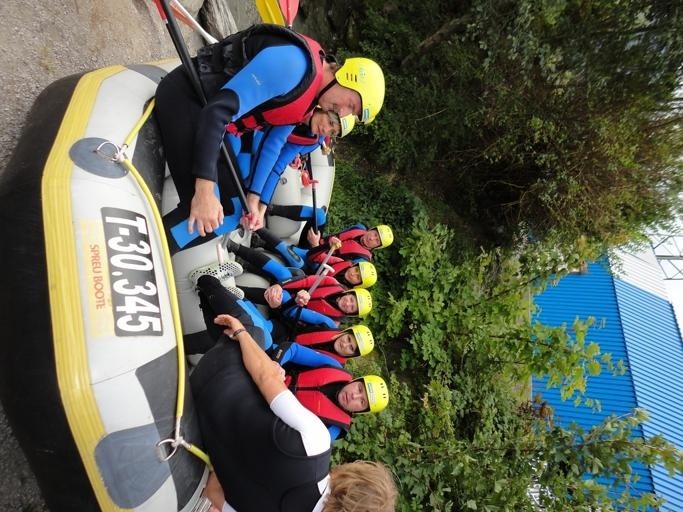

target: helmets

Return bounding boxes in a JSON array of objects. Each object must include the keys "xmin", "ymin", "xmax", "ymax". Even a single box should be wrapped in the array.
[
  {"xmin": 339, "ymin": 325, "xmax": 375, "ymax": 358},
  {"xmin": 352, "ymin": 261, "xmax": 378, "ymax": 288},
  {"xmin": 316, "ymin": 102, "xmax": 355, "ymax": 138},
  {"xmin": 348, "ymin": 375, "xmax": 389, "ymax": 414},
  {"xmin": 334, "ymin": 56, "xmax": 384, "ymax": 125},
  {"xmin": 368, "ymin": 224, "xmax": 394, "ymax": 249},
  {"xmin": 343, "ymin": 288, "xmax": 372, "ymax": 318}
]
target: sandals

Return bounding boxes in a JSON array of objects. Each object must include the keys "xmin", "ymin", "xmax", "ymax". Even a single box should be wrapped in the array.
[
  {"xmin": 188, "ymin": 261, "xmax": 243, "ymax": 287},
  {"xmin": 227, "ymin": 286, "xmax": 245, "ymax": 302}
]
